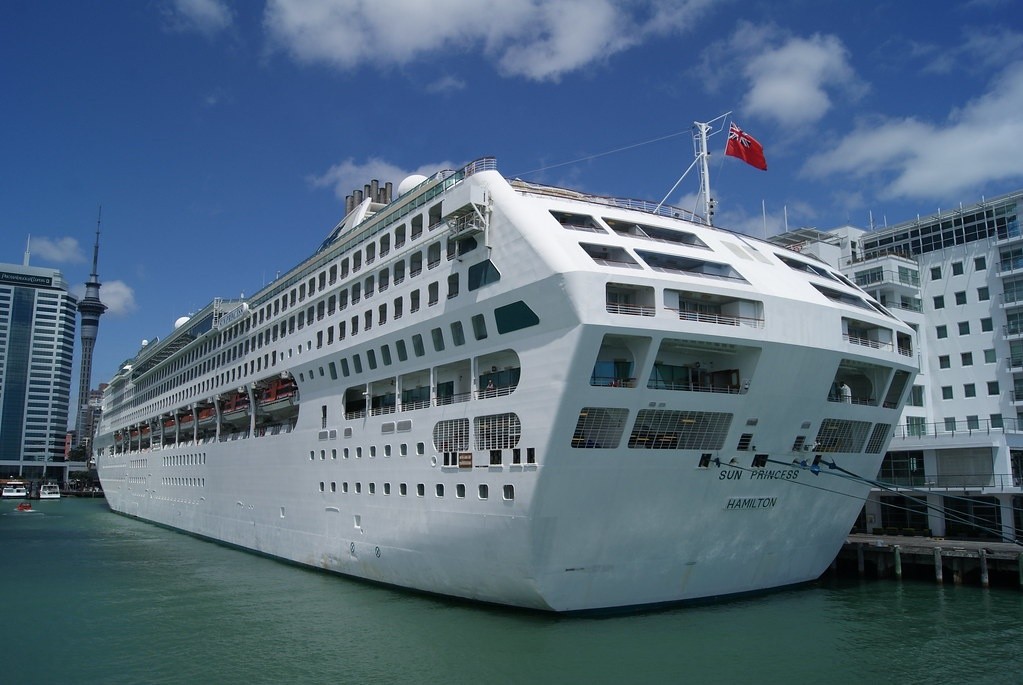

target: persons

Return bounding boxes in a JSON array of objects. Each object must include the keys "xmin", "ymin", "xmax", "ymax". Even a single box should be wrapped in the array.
[{"xmin": 486, "ymin": 380, "xmax": 494, "ymax": 389}]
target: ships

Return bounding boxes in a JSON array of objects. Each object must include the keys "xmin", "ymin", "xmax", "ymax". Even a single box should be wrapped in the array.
[{"xmin": 93, "ymin": 109, "xmax": 921, "ymax": 621}]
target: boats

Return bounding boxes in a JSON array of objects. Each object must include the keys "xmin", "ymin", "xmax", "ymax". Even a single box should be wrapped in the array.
[
  {"xmin": 197, "ymin": 408, "xmax": 218, "ymax": 430},
  {"xmin": 14, "ymin": 501, "xmax": 38, "ymax": 513},
  {"xmin": 165, "ymin": 420, "xmax": 177, "ymax": 435},
  {"xmin": 261, "ymin": 375, "xmax": 298, "ymax": 422},
  {"xmin": 114, "ymin": 433, "xmax": 122, "ymax": 447},
  {"xmin": 130, "ymin": 431, "xmax": 140, "ymax": 443},
  {"xmin": 1, "ymin": 481, "xmax": 29, "ymax": 498},
  {"xmin": 179, "ymin": 414, "xmax": 195, "ymax": 433},
  {"xmin": 142, "ymin": 427, "xmax": 150, "ymax": 442},
  {"xmin": 39, "ymin": 482, "xmax": 60, "ymax": 499},
  {"xmin": 219, "ymin": 392, "xmax": 252, "ymax": 429}
]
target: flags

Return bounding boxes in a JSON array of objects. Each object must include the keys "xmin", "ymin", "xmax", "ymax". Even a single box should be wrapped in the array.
[{"xmin": 724, "ymin": 120, "xmax": 768, "ymax": 171}]
